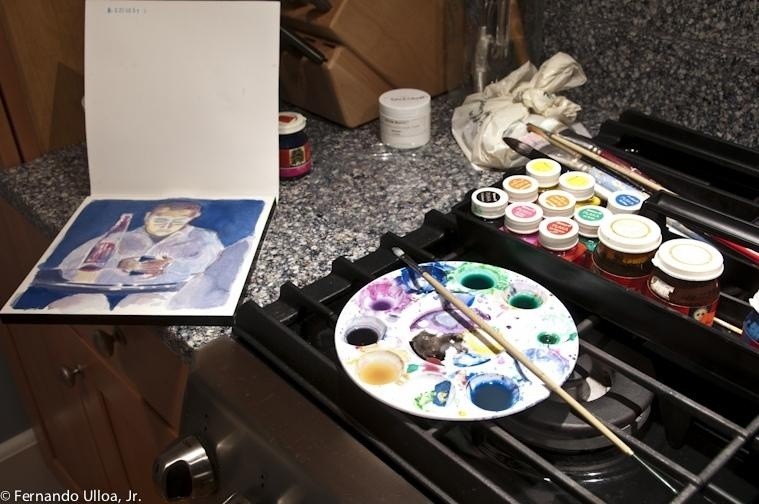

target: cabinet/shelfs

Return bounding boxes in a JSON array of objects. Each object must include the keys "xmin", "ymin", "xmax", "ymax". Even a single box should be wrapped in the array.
[
  {"xmin": 1, "ymin": 196, "xmax": 188, "ymax": 504},
  {"xmin": 0, "ymin": 0, "xmax": 85, "ymax": 172}
]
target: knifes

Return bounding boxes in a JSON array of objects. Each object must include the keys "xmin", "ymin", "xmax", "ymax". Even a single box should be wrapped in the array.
[{"xmin": 279, "ymin": 0, "xmax": 333, "ymax": 65}]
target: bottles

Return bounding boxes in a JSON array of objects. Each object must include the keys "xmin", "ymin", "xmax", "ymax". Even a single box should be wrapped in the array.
[
  {"xmin": 74, "ymin": 212, "xmax": 132, "ymax": 273},
  {"xmin": 375, "ymin": 84, "xmax": 434, "ymax": 152},
  {"xmin": 466, "ymin": 156, "xmax": 725, "ymax": 331},
  {"xmin": 276, "ymin": 108, "xmax": 316, "ymax": 181}
]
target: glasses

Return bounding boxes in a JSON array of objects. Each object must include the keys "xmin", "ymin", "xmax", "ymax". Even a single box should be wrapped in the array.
[{"xmin": 146, "ymin": 218, "xmax": 189, "ymax": 225}]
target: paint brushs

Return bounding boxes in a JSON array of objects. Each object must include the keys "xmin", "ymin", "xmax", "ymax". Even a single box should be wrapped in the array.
[
  {"xmin": 391, "ymin": 246, "xmax": 679, "ymax": 498},
  {"xmin": 501, "ymin": 123, "xmax": 759, "ymax": 267}
]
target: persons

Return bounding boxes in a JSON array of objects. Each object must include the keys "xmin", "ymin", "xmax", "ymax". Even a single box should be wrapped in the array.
[{"xmin": 43, "ymin": 200, "xmax": 252, "ymax": 313}]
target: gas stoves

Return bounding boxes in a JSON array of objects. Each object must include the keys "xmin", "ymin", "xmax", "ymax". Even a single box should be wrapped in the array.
[{"xmin": 150, "ymin": 105, "xmax": 758, "ymax": 502}]
